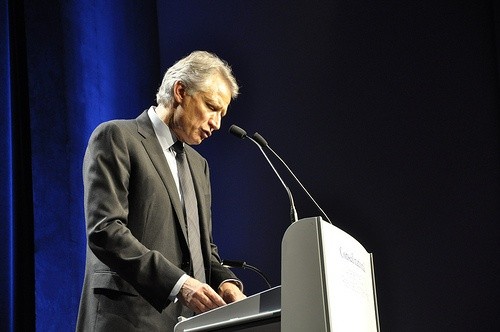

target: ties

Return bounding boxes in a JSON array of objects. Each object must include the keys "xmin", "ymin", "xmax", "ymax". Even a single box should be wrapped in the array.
[{"xmin": 170, "ymin": 140, "xmax": 206, "ymax": 284}]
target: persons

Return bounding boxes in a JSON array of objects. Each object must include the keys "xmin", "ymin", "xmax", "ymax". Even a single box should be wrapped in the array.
[{"xmin": 76, "ymin": 51, "xmax": 248, "ymax": 332}]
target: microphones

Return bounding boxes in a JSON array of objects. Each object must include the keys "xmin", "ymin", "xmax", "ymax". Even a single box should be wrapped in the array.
[
  {"xmin": 221, "ymin": 260, "xmax": 272, "ymax": 288},
  {"xmin": 229, "ymin": 125, "xmax": 332, "ymax": 223}
]
